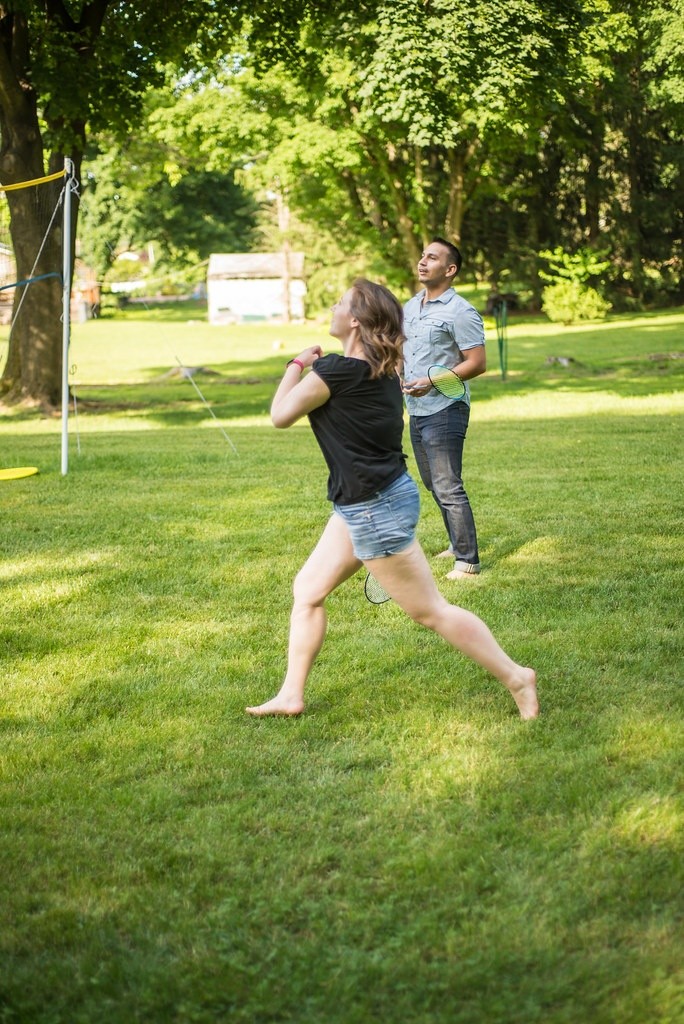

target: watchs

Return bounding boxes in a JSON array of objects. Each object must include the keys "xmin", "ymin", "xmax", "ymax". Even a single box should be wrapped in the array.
[{"xmin": 286, "ymin": 359, "xmax": 305, "ymax": 375}]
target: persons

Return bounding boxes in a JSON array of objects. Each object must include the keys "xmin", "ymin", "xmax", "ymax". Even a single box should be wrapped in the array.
[
  {"xmin": 396, "ymin": 237, "xmax": 488, "ymax": 581},
  {"xmin": 246, "ymin": 277, "xmax": 539, "ymax": 724}
]
